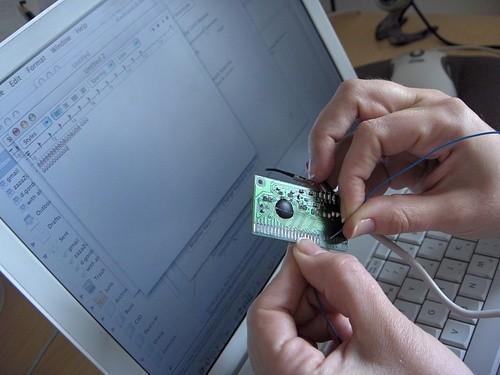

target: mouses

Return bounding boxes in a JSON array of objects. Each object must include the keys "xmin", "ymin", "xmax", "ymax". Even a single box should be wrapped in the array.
[{"xmin": 391, "ymin": 50, "xmax": 458, "ymax": 97}]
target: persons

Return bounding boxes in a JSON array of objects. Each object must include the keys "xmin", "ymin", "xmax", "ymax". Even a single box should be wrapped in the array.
[{"xmin": 245, "ymin": 79, "xmax": 500, "ymax": 375}]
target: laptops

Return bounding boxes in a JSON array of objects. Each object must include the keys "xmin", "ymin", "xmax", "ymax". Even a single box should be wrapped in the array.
[{"xmin": 0, "ymin": 0, "xmax": 500, "ymax": 375}]
